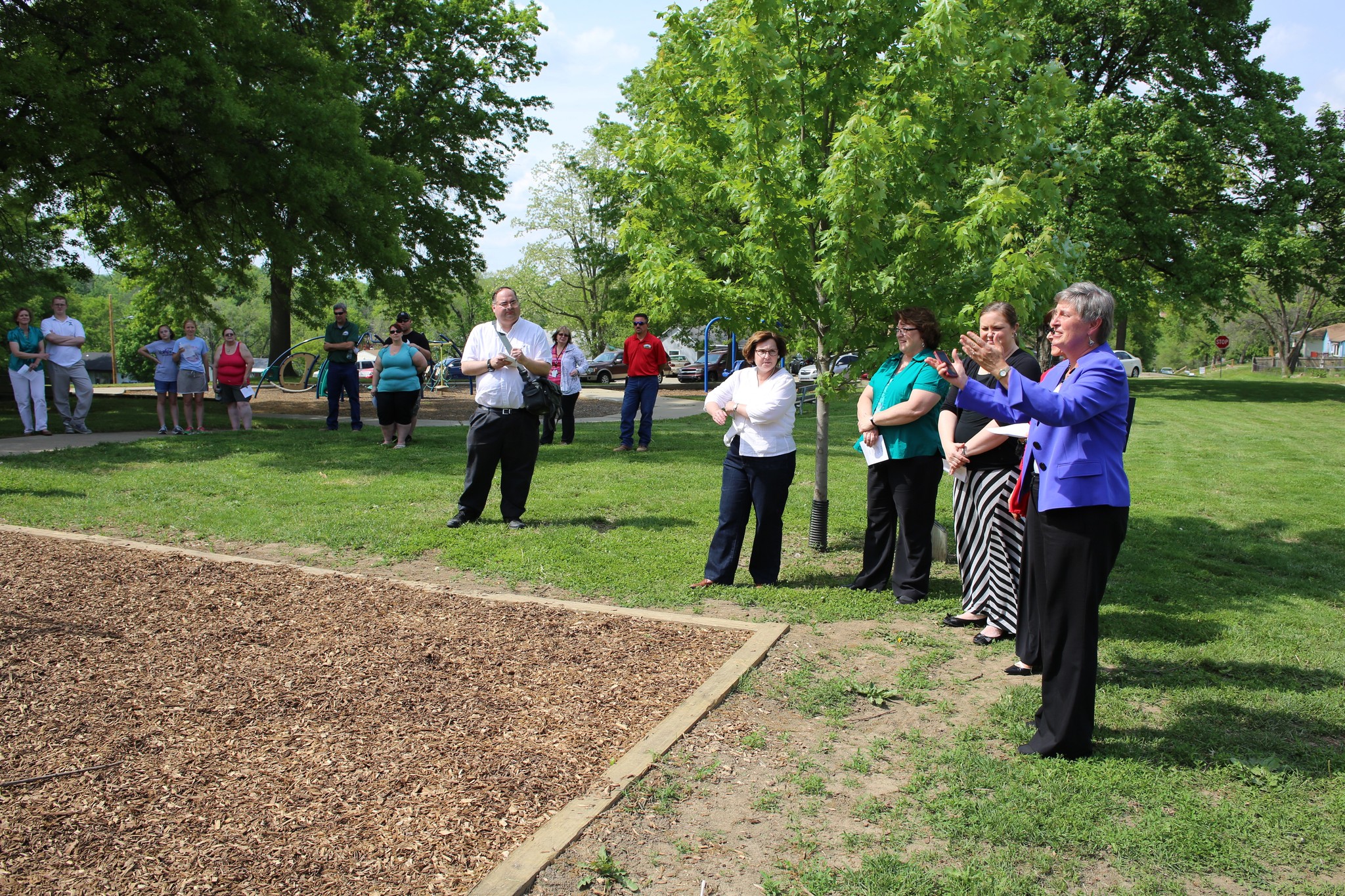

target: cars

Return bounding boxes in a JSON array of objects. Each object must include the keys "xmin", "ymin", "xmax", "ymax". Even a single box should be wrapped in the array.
[
  {"xmin": 1159, "ymin": 368, "xmax": 1174, "ymax": 375},
  {"xmin": 1109, "ymin": 350, "xmax": 1142, "ymax": 378},
  {"xmin": 356, "ymin": 360, "xmax": 377, "ymax": 380},
  {"xmin": 797, "ymin": 353, "xmax": 859, "ymax": 383},
  {"xmin": 661, "ymin": 355, "xmax": 691, "ymax": 377},
  {"xmin": 789, "ymin": 351, "xmax": 817, "ymax": 374},
  {"xmin": 431, "ymin": 358, "xmax": 477, "ymax": 380},
  {"xmin": 578, "ymin": 350, "xmax": 663, "ymax": 385}
]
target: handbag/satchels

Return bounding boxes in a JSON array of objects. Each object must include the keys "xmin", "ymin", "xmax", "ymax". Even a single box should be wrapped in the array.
[{"xmin": 522, "ymin": 377, "xmax": 563, "ymax": 432}]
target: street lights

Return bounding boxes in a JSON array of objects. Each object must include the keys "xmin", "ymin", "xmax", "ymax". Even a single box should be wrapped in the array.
[{"xmin": 107, "ymin": 294, "xmax": 134, "ymax": 384}]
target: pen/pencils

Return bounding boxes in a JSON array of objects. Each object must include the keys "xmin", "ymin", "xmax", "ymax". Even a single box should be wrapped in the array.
[{"xmin": 521, "ymin": 345, "xmax": 526, "ymax": 349}]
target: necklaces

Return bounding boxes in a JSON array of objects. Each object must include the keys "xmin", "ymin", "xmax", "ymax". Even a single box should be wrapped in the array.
[
  {"xmin": 758, "ymin": 366, "xmax": 776, "ymax": 388},
  {"xmin": 1061, "ymin": 363, "xmax": 1077, "ymax": 384},
  {"xmin": 227, "ymin": 349, "xmax": 230, "ymax": 352}
]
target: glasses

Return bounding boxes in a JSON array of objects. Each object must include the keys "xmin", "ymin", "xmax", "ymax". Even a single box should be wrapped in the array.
[
  {"xmin": 334, "ymin": 311, "xmax": 345, "ymax": 315},
  {"xmin": 894, "ymin": 328, "xmax": 917, "ymax": 334},
  {"xmin": 497, "ymin": 301, "xmax": 520, "ymax": 309},
  {"xmin": 53, "ymin": 303, "xmax": 66, "ymax": 306},
  {"xmin": 558, "ymin": 333, "xmax": 569, "ymax": 337},
  {"xmin": 1047, "ymin": 328, "xmax": 1055, "ymax": 335},
  {"xmin": 397, "ymin": 317, "xmax": 409, "ymax": 323},
  {"xmin": 633, "ymin": 321, "xmax": 647, "ymax": 326},
  {"xmin": 755, "ymin": 349, "xmax": 780, "ymax": 356},
  {"xmin": 225, "ymin": 334, "xmax": 235, "ymax": 337},
  {"xmin": 390, "ymin": 329, "xmax": 401, "ymax": 334}
]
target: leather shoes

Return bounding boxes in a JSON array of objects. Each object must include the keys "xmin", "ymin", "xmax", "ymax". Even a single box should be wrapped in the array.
[
  {"xmin": 1004, "ymin": 661, "xmax": 1032, "ymax": 675},
  {"xmin": 841, "ymin": 584, "xmax": 868, "ymax": 591},
  {"xmin": 894, "ymin": 596, "xmax": 918, "ymax": 605},
  {"xmin": 755, "ymin": 582, "xmax": 764, "ymax": 589},
  {"xmin": 690, "ymin": 578, "xmax": 717, "ymax": 588},
  {"xmin": 972, "ymin": 626, "xmax": 1016, "ymax": 646},
  {"xmin": 942, "ymin": 612, "xmax": 988, "ymax": 627}
]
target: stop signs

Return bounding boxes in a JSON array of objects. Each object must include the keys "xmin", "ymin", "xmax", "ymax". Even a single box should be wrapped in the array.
[{"xmin": 1215, "ymin": 335, "xmax": 1229, "ymax": 349}]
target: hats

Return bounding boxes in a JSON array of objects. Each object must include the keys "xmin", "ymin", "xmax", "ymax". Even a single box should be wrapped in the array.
[{"xmin": 396, "ymin": 311, "xmax": 410, "ymax": 321}]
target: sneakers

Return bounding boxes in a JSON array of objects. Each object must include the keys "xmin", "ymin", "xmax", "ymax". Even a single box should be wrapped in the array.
[
  {"xmin": 68, "ymin": 423, "xmax": 92, "ymax": 434},
  {"xmin": 196, "ymin": 426, "xmax": 208, "ymax": 433},
  {"xmin": 613, "ymin": 444, "xmax": 633, "ymax": 451},
  {"xmin": 636, "ymin": 445, "xmax": 648, "ymax": 452},
  {"xmin": 503, "ymin": 517, "xmax": 527, "ymax": 529},
  {"xmin": 64, "ymin": 425, "xmax": 75, "ymax": 434},
  {"xmin": 172, "ymin": 425, "xmax": 184, "ymax": 435},
  {"xmin": 446, "ymin": 511, "xmax": 481, "ymax": 527},
  {"xmin": 158, "ymin": 425, "xmax": 168, "ymax": 434},
  {"xmin": 183, "ymin": 426, "xmax": 195, "ymax": 435}
]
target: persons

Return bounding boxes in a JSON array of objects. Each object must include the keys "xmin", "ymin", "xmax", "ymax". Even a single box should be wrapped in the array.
[
  {"xmin": 7, "ymin": 307, "xmax": 52, "ymax": 436},
  {"xmin": 690, "ymin": 331, "xmax": 796, "ymax": 589},
  {"xmin": 212, "ymin": 327, "xmax": 255, "ymax": 431},
  {"xmin": 540, "ymin": 326, "xmax": 589, "ymax": 445},
  {"xmin": 924, "ymin": 282, "xmax": 1131, "ymax": 760},
  {"xmin": 40, "ymin": 296, "xmax": 94, "ymax": 434},
  {"xmin": 381, "ymin": 311, "xmax": 432, "ymax": 442},
  {"xmin": 839, "ymin": 306, "xmax": 951, "ymax": 605},
  {"xmin": 370, "ymin": 323, "xmax": 428, "ymax": 449},
  {"xmin": 319, "ymin": 303, "xmax": 364, "ymax": 432},
  {"xmin": 614, "ymin": 313, "xmax": 667, "ymax": 452},
  {"xmin": 172, "ymin": 320, "xmax": 210, "ymax": 435},
  {"xmin": 447, "ymin": 286, "xmax": 552, "ymax": 528},
  {"xmin": 138, "ymin": 324, "xmax": 185, "ymax": 434}
]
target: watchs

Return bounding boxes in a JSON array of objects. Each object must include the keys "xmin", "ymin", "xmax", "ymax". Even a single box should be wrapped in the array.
[
  {"xmin": 869, "ymin": 415, "xmax": 877, "ymax": 427},
  {"xmin": 487, "ymin": 359, "xmax": 495, "ymax": 372},
  {"xmin": 957, "ymin": 447, "xmax": 968, "ymax": 458},
  {"xmin": 995, "ymin": 366, "xmax": 1012, "ymax": 380},
  {"xmin": 732, "ymin": 403, "xmax": 739, "ymax": 413}
]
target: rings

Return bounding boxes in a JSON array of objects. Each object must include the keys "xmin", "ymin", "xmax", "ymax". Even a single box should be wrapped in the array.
[{"xmin": 972, "ymin": 351, "xmax": 976, "ymax": 356}]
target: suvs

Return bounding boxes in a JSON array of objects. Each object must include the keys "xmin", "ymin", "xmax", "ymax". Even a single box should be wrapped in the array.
[{"xmin": 677, "ymin": 348, "xmax": 746, "ymax": 383}]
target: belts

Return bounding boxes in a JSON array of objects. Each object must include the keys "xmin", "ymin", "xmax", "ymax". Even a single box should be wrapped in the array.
[{"xmin": 479, "ymin": 405, "xmax": 527, "ymax": 415}]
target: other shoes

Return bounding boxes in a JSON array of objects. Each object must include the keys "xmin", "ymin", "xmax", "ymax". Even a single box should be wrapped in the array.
[
  {"xmin": 318, "ymin": 427, "xmax": 335, "ymax": 431},
  {"xmin": 40, "ymin": 429, "xmax": 52, "ymax": 436},
  {"xmin": 351, "ymin": 429, "xmax": 359, "ymax": 432},
  {"xmin": 375, "ymin": 435, "xmax": 413, "ymax": 450},
  {"xmin": 1026, "ymin": 721, "xmax": 1037, "ymax": 731},
  {"xmin": 24, "ymin": 430, "xmax": 37, "ymax": 436},
  {"xmin": 1018, "ymin": 743, "xmax": 1035, "ymax": 755}
]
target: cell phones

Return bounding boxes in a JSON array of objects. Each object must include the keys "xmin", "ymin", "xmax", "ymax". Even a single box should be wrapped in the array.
[{"xmin": 934, "ymin": 351, "xmax": 958, "ymax": 377}]
